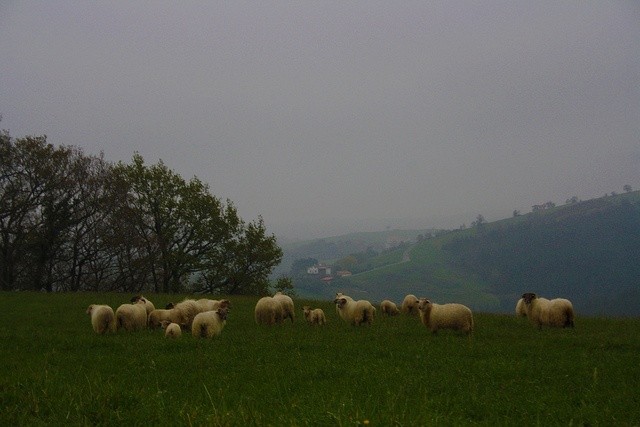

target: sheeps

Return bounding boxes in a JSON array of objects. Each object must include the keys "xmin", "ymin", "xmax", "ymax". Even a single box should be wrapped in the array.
[
  {"xmin": 334, "ymin": 292, "xmax": 377, "ymax": 328},
  {"xmin": 414, "ymin": 297, "xmax": 474, "ymax": 338},
  {"xmin": 514, "ymin": 292, "xmax": 546, "ymax": 326},
  {"xmin": 254, "ymin": 291, "xmax": 296, "ymax": 328},
  {"xmin": 84, "ymin": 302, "xmax": 115, "ymax": 337},
  {"xmin": 128, "ymin": 294, "xmax": 233, "ymax": 342},
  {"xmin": 300, "ymin": 305, "xmax": 330, "ymax": 328},
  {"xmin": 380, "ymin": 299, "xmax": 400, "ymax": 320},
  {"xmin": 401, "ymin": 294, "xmax": 419, "ymax": 317},
  {"xmin": 115, "ymin": 298, "xmax": 148, "ymax": 335},
  {"xmin": 521, "ymin": 292, "xmax": 574, "ymax": 332}
]
